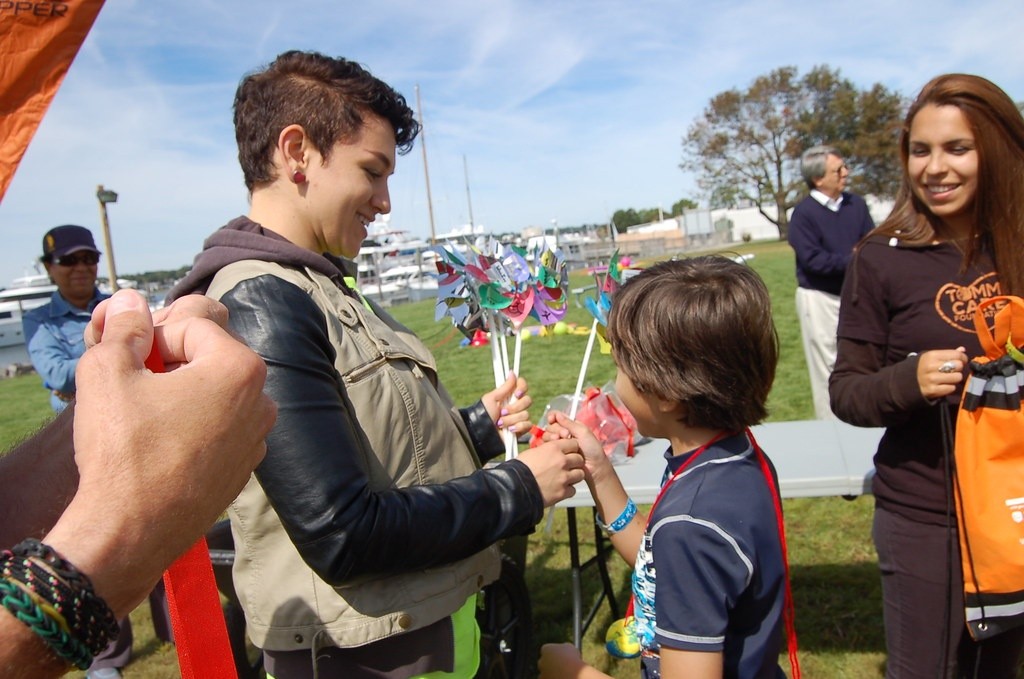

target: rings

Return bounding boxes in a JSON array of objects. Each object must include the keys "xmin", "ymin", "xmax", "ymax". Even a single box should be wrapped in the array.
[{"xmin": 938, "ymin": 360, "xmax": 957, "ymax": 374}]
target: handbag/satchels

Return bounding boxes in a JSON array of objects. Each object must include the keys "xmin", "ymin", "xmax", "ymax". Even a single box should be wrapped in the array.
[{"xmin": 948, "ymin": 283, "xmax": 1024, "ymax": 641}]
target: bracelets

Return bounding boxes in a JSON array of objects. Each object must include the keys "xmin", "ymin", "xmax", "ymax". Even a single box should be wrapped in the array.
[
  {"xmin": 0, "ymin": 536, "xmax": 124, "ymax": 670},
  {"xmin": 594, "ymin": 497, "xmax": 637, "ymax": 540}
]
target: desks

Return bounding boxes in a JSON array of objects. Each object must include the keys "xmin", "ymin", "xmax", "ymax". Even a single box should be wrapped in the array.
[{"xmin": 546, "ymin": 418, "xmax": 887, "ymax": 654}]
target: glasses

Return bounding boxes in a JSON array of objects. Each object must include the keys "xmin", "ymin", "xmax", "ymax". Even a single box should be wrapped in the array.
[
  {"xmin": 827, "ymin": 160, "xmax": 849, "ymax": 175},
  {"xmin": 40, "ymin": 256, "xmax": 100, "ymax": 266}
]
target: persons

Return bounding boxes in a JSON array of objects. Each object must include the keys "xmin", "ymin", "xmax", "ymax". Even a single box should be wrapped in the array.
[
  {"xmin": 786, "ymin": 143, "xmax": 878, "ymax": 502},
  {"xmin": 535, "ymin": 252, "xmax": 789, "ymax": 679},
  {"xmin": 827, "ymin": 71, "xmax": 1024, "ymax": 679},
  {"xmin": 161, "ymin": 48, "xmax": 588, "ymax": 678},
  {"xmin": 21, "ymin": 225, "xmax": 117, "ymax": 422},
  {"xmin": 0, "ymin": 287, "xmax": 279, "ymax": 679}
]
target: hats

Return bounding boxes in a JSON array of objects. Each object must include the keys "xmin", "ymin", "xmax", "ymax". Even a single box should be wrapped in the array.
[{"xmin": 43, "ymin": 225, "xmax": 102, "ymax": 257}]
